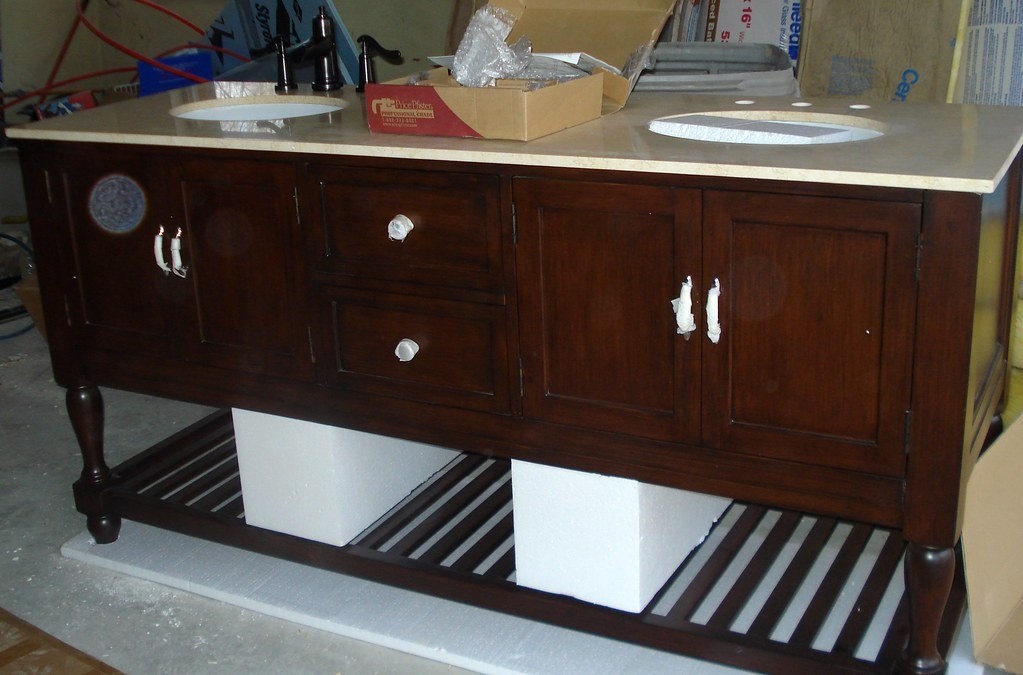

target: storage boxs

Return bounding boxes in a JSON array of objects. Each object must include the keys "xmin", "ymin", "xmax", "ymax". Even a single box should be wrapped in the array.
[
  {"xmin": 365, "ymin": 0, "xmax": 676, "ymax": 142},
  {"xmin": 231, "ymin": 407, "xmax": 463, "ymax": 546},
  {"xmin": 511, "ymin": 459, "xmax": 734, "ymax": 615}
]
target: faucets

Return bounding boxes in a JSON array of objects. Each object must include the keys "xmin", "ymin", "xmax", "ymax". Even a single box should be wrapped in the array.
[{"xmin": 277, "ymin": 6, "xmax": 344, "ymax": 92}]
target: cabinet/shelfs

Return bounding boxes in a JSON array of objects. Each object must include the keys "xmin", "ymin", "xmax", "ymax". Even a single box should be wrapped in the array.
[{"xmin": 3, "ymin": 79, "xmax": 1023, "ymax": 675}]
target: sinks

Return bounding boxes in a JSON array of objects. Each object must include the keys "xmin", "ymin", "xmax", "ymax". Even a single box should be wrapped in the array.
[
  {"xmin": 646, "ymin": 110, "xmax": 889, "ymax": 145},
  {"xmin": 168, "ymin": 95, "xmax": 350, "ymax": 122}
]
